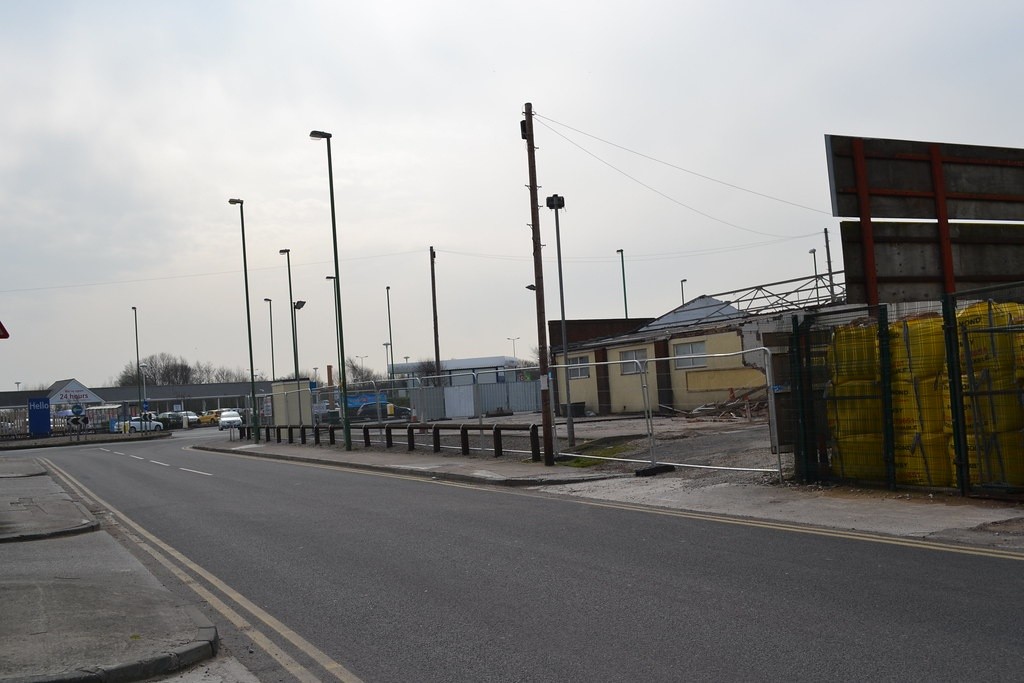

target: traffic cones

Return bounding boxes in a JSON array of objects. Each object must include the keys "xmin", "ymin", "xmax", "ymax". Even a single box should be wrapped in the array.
[{"xmin": 410, "ymin": 403, "xmax": 420, "ymax": 423}]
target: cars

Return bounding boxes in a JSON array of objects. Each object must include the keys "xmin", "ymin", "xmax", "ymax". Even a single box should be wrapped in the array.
[
  {"xmin": 217, "ymin": 411, "xmax": 243, "ymax": 431},
  {"xmin": 136, "ymin": 406, "xmax": 259, "ymax": 430},
  {"xmin": 313, "ymin": 404, "xmax": 340, "ymax": 424},
  {"xmin": 113, "ymin": 416, "xmax": 164, "ymax": 434},
  {"xmin": 357, "ymin": 401, "xmax": 411, "ymax": 422}
]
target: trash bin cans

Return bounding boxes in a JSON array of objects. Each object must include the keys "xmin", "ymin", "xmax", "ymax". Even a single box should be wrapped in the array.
[{"xmin": 561, "ymin": 401, "xmax": 585, "ymax": 417}]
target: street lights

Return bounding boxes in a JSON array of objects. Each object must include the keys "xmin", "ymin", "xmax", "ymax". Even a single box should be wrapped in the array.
[
  {"xmin": 278, "ymin": 247, "xmax": 297, "ymax": 380},
  {"xmin": 403, "ymin": 356, "xmax": 410, "ymax": 363},
  {"xmin": 130, "ymin": 305, "xmax": 142, "ymax": 413},
  {"xmin": 264, "ymin": 297, "xmax": 275, "ymax": 383},
  {"xmin": 14, "ymin": 381, "xmax": 22, "ymax": 391},
  {"xmin": 140, "ymin": 362, "xmax": 148, "ymax": 412},
  {"xmin": 226, "ymin": 196, "xmax": 260, "ymax": 426},
  {"xmin": 615, "ymin": 248, "xmax": 628, "ymax": 319},
  {"xmin": 323, "ymin": 274, "xmax": 344, "ymax": 424},
  {"xmin": 292, "ymin": 301, "xmax": 308, "ymax": 424},
  {"xmin": 308, "ymin": 128, "xmax": 354, "ymax": 450},
  {"xmin": 506, "ymin": 337, "xmax": 521, "ymax": 381},
  {"xmin": 680, "ymin": 279, "xmax": 687, "ymax": 306},
  {"xmin": 545, "ymin": 193, "xmax": 577, "ymax": 447},
  {"xmin": 525, "ymin": 284, "xmax": 556, "ymax": 466},
  {"xmin": 312, "ymin": 367, "xmax": 319, "ymax": 388},
  {"xmin": 355, "ymin": 355, "xmax": 369, "ymax": 381},
  {"xmin": 383, "ymin": 341, "xmax": 391, "ymax": 379},
  {"xmin": 809, "ymin": 248, "xmax": 820, "ymax": 305},
  {"xmin": 385, "ymin": 286, "xmax": 396, "ymax": 388}
]
